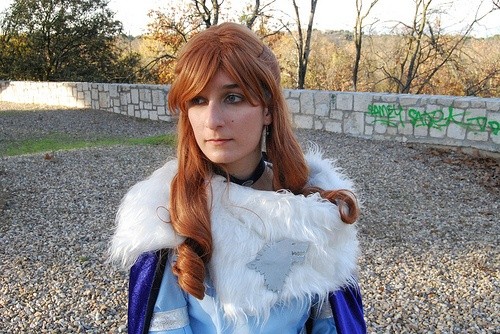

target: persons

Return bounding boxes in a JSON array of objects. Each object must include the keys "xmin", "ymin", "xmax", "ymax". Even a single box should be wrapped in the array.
[{"xmin": 120, "ymin": 22, "xmax": 371, "ymax": 334}]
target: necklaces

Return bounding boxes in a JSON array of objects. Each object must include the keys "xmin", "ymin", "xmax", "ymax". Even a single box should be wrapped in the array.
[{"xmin": 210, "ymin": 155, "xmax": 268, "ymax": 189}]
